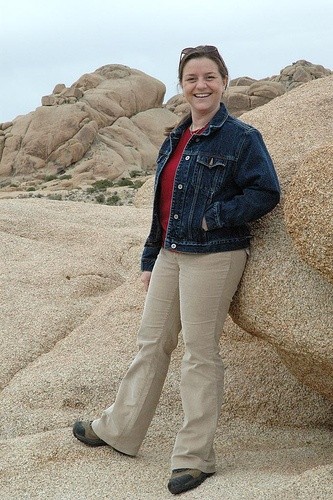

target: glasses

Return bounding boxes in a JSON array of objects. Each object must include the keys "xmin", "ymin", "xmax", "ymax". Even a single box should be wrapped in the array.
[{"xmin": 178, "ymin": 45, "xmax": 225, "ymax": 66}]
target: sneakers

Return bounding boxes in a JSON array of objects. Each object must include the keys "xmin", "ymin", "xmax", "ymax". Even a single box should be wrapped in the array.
[
  {"xmin": 168, "ymin": 469, "xmax": 215, "ymax": 495},
  {"xmin": 72, "ymin": 420, "xmax": 134, "ymax": 456}
]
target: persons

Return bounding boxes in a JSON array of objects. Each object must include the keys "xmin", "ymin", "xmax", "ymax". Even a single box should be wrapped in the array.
[{"xmin": 72, "ymin": 45, "xmax": 282, "ymax": 494}]
